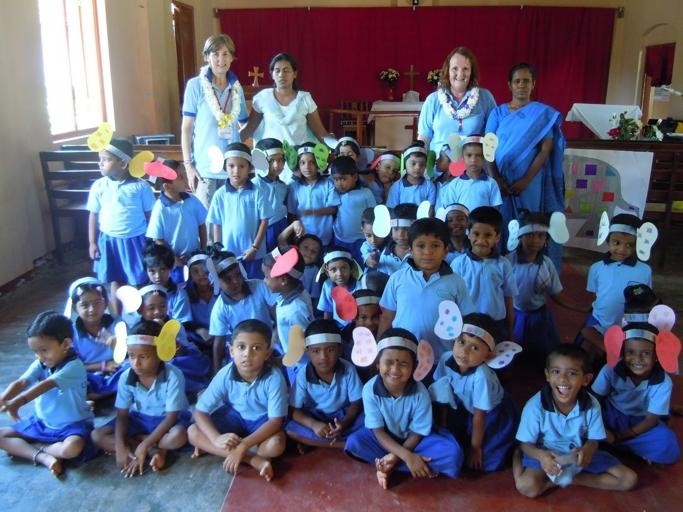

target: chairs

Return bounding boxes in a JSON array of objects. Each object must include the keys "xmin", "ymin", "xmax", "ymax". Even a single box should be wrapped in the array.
[{"xmin": 39, "ymin": 150, "xmax": 103, "ymax": 261}]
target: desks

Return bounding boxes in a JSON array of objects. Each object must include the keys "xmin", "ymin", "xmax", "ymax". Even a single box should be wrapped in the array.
[{"xmin": 367, "ymin": 99, "xmax": 427, "ymax": 149}]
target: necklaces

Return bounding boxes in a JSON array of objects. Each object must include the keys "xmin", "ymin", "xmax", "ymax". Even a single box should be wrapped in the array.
[
  {"xmin": 200, "ymin": 65, "xmax": 241, "ymax": 128},
  {"xmin": 438, "ymin": 85, "xmax": 480, "ymax": 119}
]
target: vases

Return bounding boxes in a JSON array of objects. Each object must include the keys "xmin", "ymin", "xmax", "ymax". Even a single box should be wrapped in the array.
[{"xmin": 382, "ymin": 86, "xmax": 398, "ymax": 100}]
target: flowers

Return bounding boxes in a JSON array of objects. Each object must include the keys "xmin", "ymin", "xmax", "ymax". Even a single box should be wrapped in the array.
[
  {"xmin": 428, "ymin": 69, "xmax": 443, "ymax": 83},
  {"xmin": 377, "ymin": 67, "xmax": 403, "ymax": 87}
]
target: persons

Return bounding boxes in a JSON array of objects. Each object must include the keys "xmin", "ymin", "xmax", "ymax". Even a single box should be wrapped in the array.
[
  {"xmin": 418, "ymin": 45, "xmax": 498, "ymax": 177},
  {"xmin": 240, "ymin": 52, "xmax": 331, "ymax": 186},
  {"xmin": 0, "ymin": 133, "xmax": 681, "ymax": 499},
  {"xmin": 179, "ymin": 33, "xmax": 250, "ymax": 209},
  {"xmin": 486, "ymin": 62, "xmax": 566, "ymax": 275}
]
